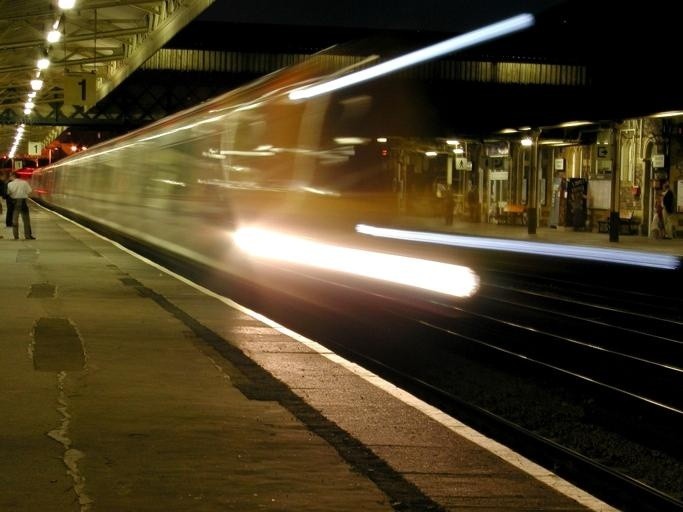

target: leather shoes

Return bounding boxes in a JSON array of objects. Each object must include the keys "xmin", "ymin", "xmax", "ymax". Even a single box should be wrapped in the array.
[{"xmin": 26, "ymin": 235, "xmax": 36, "ymax": 239}]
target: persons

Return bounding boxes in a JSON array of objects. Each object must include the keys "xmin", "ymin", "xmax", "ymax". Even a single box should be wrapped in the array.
[
  {"xmin": 0, "ymin": 171, "xmax": 39, "ymax": 240},
  {"xmin": 660, "ymin": 183, "xmax": 675, "ymax": 242},
  {"xmin": 468, "ymin": 184, "xmax": 479, "ymax": 219},
  {"xmin": 443, "ymin": 183, "xmax": 456, "ymax": 224}
]
